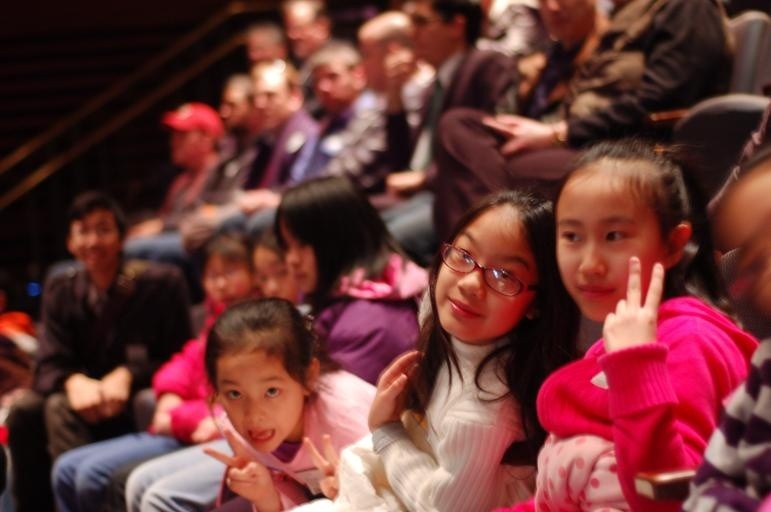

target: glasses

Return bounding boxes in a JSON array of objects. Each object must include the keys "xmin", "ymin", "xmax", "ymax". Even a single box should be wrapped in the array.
[{"xmin": 439, "ymin": 240, "xmax": 537, "ymax": 298}]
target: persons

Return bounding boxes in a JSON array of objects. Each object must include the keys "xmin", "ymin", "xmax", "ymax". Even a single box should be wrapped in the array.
[{"xmin": 0, "ymin": 1, "xmax": 771, "ymax": 512}]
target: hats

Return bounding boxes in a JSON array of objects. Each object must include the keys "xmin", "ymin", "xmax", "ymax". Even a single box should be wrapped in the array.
[{"xmin": 162, "ymin": 101, "xmax": 224, "ymax": 136}]
